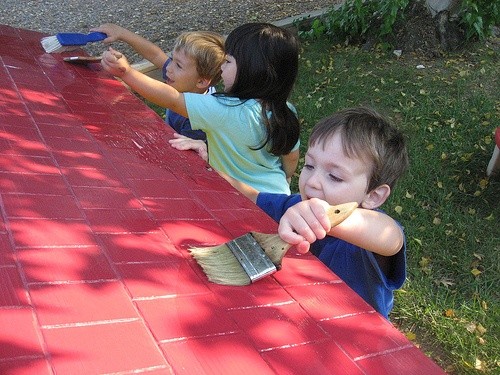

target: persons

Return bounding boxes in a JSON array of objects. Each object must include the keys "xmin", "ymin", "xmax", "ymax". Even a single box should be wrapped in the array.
[
  {"xmin": 168, "ymin": 106, "xmax": 409, "ymax": 320},
  {"xmin": 88, "ymin": 21, "xmax": 228, "ymax": 164},
  {"xmin": 99, "ymin": 22, "xmax": 300, "ymax": 197}
]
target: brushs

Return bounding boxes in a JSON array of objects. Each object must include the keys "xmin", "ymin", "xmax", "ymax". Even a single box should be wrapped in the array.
[
  {"xmin": 40, "ymin": 31, "xmax": 109, "ymax": 54},
  {"xmin": 186, "ymin": 201, "xmax": 360, "ymax": 288},
  {"xmin": 62, "ymin": 53, "xmax": 122, "ymax": 63}
]
